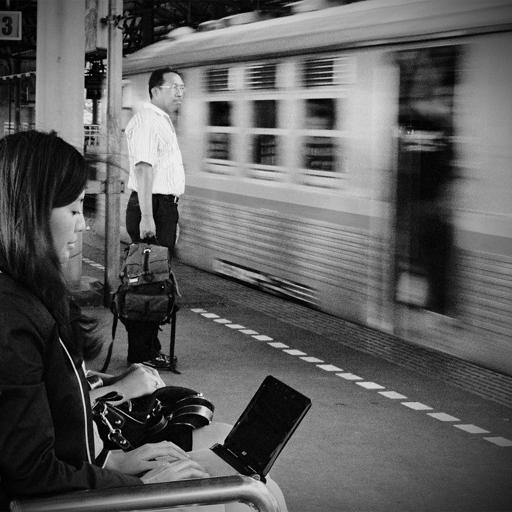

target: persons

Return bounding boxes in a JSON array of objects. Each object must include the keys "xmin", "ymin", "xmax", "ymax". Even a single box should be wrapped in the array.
[
  {"xmin": 0, "ymin": 128, "xmax": 289, "ymax": 512},
  {"xmin": 79, "ymin": 360, "xmax": 235, "ymax": 450},
  {"xmin": 123, "ymin": 68, "xmax": 188, "ymax": 372}
]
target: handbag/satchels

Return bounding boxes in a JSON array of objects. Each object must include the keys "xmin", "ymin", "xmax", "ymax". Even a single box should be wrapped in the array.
[{"xmin": 93, "ymin": 378, "xmax": 217, "ymax": 452}]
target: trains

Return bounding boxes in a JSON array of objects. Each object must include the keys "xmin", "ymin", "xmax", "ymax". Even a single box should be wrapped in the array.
[{"xmin": 0, "ymin": 0, "xmax": 512, "ymax": 374}]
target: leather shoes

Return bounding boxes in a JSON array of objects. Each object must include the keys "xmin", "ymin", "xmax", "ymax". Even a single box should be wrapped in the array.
[{"xmin": 127, "ymin": 352, "xmax": 178, "ymax": 371}]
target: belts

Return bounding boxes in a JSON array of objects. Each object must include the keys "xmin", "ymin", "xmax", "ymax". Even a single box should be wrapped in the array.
[{"xmin": 130, "ymin": 191, "xmax": 178, "ymax": 205}]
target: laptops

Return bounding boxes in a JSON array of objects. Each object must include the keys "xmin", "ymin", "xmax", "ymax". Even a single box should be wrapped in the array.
[{"xmin": 154, "ymin": 374, "xmax": 313, "ymax": 485}]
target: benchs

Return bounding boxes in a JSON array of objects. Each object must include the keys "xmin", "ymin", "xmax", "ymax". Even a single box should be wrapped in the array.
[{"xmin": 6, "ymin": 472, "xmax": 284, "ymax": 512}]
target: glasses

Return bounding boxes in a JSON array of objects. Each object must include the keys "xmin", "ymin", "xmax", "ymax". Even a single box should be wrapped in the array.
[{"xmin": 157, "ymin": 84, "xmax": 185, "ymax": 92}]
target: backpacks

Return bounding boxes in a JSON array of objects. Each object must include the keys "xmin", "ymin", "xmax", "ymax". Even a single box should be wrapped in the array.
[{"xmin": 109, "ymin": 232, "xmax": 186, "ymax": 329}]
